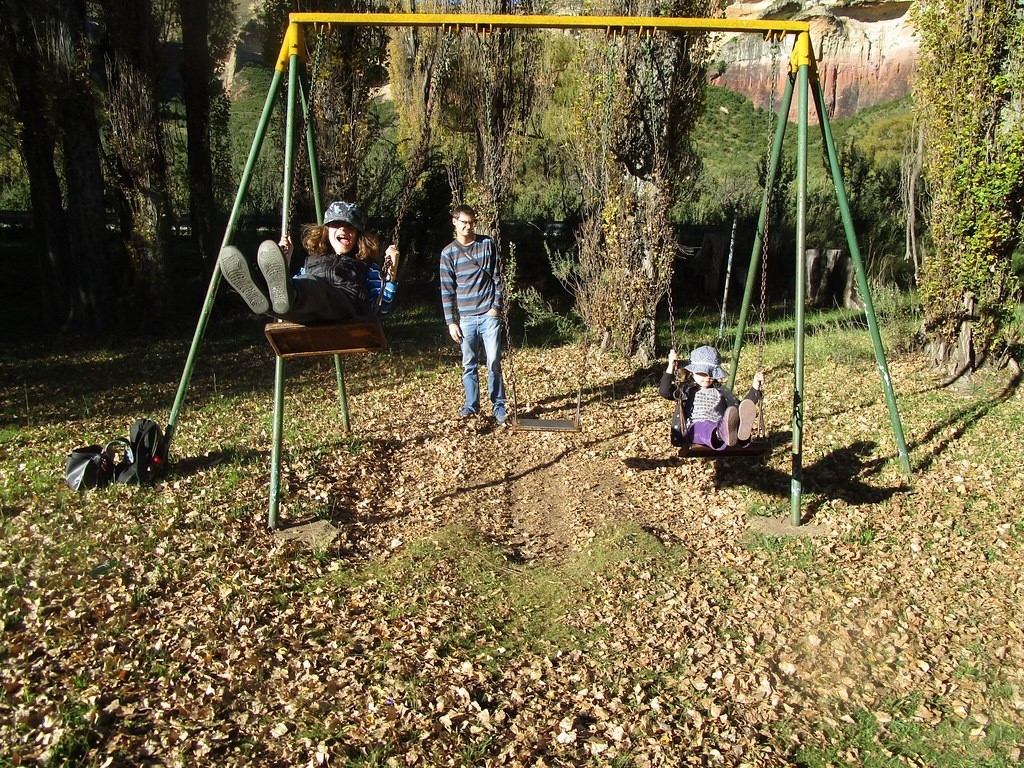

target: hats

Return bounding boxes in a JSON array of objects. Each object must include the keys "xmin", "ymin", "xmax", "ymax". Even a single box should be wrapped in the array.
[
  {"xmin": 323, "ymin": 199, "xmax": 367, "ymax": 233},
  {"xmin": 682, "ymin": 346, "xmax": 730, "ymax": 380}
]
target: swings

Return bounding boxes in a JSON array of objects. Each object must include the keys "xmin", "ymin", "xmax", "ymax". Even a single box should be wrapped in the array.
[
  {"xmin": 481, "ymin": 28, "xmax": 619, "ymax": 432},
  {"xmin": 648, "ymin": 29, "xmax": 773, "ymax": 459},
  {"xmin": 264, "ymin": 27, "xmax": 456, "ymax": 358}
]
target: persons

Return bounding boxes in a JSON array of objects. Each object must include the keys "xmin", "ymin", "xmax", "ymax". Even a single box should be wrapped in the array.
[
  {"xmin": 660, "ymin": 346, "xmax": 765, "ymax": 450},
  {"xmin": 440, "ymin": 205, "xmax": 508, "ymax": 423},
  {"xmin": 220, "ymin": 201, "xmax": 398, "ymax": 326}
]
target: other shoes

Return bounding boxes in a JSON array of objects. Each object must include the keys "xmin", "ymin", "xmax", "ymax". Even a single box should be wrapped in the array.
[
  {"xmin": 220, "ymin": 245, "xmax": 270, "ymax": 315},
  {"xmin": 257, "ymin": 239, "xmax": 290, "ymax": 315}
]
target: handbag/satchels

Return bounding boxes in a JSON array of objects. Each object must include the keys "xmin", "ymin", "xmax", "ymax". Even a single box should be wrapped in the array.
[{"xmin": 64, "ymin": 437, "xmax": 134, "ymax": 494}]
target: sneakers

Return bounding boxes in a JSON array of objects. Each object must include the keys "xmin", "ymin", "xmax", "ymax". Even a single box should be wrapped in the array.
[
  {"xmin": 716, "ymin": 406, "xmax": 739, "ymax": 447},
  {"xmin": 737, "ymin": 399, "xmax": 756, "ymax": 441},
  {"xmin": 456, "ymin": 408, "xmax": 480, "ymax": 418},
  {"xmin": 492, "ymin": 405, "xmax": 508, "ymax": 424}
]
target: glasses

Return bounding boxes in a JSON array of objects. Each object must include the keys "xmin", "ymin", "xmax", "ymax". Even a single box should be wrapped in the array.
[{"xmin": 457, "ymin": 219, "xmax": 474, "ymax": 225}]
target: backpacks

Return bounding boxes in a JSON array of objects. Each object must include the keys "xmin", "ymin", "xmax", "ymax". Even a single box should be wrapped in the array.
[{"xmin": 112, "ymin": 418, "xmax": 169, "ymax": 487}]
